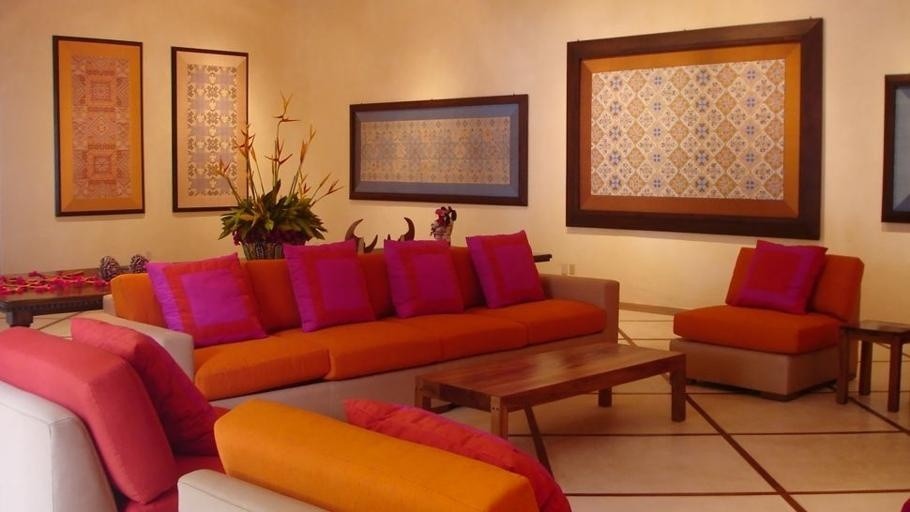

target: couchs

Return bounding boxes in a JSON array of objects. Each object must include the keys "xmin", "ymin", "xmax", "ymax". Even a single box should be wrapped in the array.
[{"xmin": 68, "ymin": 265, "xmax": 622, "ymax": 429}]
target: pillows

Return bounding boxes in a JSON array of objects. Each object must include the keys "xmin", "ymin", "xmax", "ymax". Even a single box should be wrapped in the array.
[
  {"xmin": 462, "ymin": 228, "xmax": 549, "ymax": 311},
  {"xmin": 727, "ymin": 238, "xmax": 831, "ymax": 317},
  {"xmin": 65, "ymin": 314, "xmax": 221, "ymax": 448},
  {"xmin": 281, "ymin": 236, "xmax": 379, "ymax": 334},
  {"xmin": 340, "ymin": 395, "xmax": 575, "ymax": 512},
  {"xmin": 379, "ymin": 235, "xmax": 468, "ymax": 319},
  {"xmin": 141, "ymin": 251, "xmax": 269, "ymax": 350}
]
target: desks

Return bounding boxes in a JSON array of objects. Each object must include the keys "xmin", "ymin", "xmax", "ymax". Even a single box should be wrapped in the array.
[
  {"xmin": 833, "ymin": 315, "xmax": 910, "ymax": 413},
  {"xmin": 0, "ymin": 265, "xmax": 136, "ymax": 332}
]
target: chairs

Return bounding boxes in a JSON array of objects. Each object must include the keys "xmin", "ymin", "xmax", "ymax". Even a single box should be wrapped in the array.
[
  {"xmin": 172, "ymin": 394, "xmax": 546, "ymax": 511},
  {"xmin": 666, "ymin": 244, "xmax": 867, "ymax": 403},
  {"xmin": 0, "ymin": 326, "xmax": 231, "ymax": 511}
]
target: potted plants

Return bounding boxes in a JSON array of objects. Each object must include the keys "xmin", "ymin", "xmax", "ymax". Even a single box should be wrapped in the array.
[{"xmin": 207, "ymin": 84, "xmax": 345, "ymax": 265}]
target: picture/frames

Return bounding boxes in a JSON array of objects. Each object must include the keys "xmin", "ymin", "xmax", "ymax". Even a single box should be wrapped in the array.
[
  {"xmin": 564, "ymin": 18, "xmax": 825, "ymax": 244},
  {"xmin": 343, "ymin": 93, "xmax": 529, "ymax": 208},
  {"xmin": 168, "ymin": 44, "xmax": 251, "ymax": 213},
  {"xmin": 50, "ymin": 33, "xmax": 146, "ymax": 218},
  {"xmin": 880, "ymin": 72, "xmax": 910, "ymax": 225}
]
